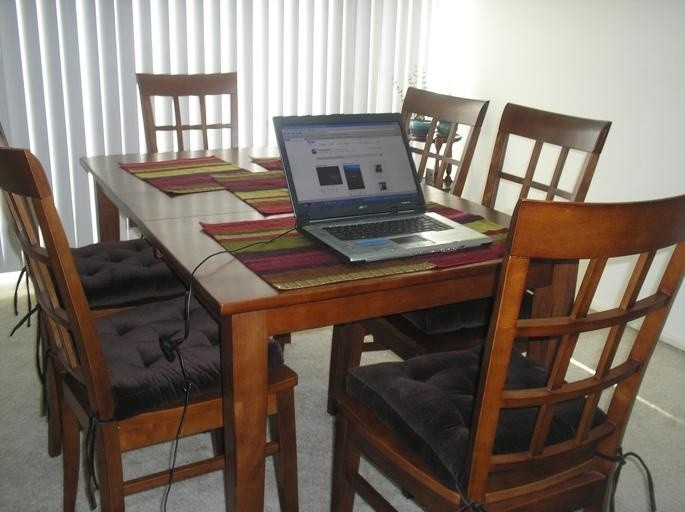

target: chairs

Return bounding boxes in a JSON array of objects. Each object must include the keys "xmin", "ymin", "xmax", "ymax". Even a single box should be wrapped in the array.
[
  {"xmin": 327, "ymin": 103, "xmax": 614, "ymax": 411},
  {"xmin": 128, "ymin": 71, "xmax": 246, "ymax": 154},
  {"xmin": 336, "ymin": 193, "xmax": 684, "ymax": 510},
  {"xmin": 1, "ymin": 124, "xmax": 187, "ymax": 461},
  {"xmin": 390, "ymin": 85, "xmax": 493, "ymax": 198},
  {"xmin": 0, "ymin": 145, "xmax": 301, "ymax": 512}
]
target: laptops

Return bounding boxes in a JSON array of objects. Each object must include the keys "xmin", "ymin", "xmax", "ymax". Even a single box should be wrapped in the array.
[{"xmin": 273, "ymin": 112, "xmax": 493, "ymax": 265}]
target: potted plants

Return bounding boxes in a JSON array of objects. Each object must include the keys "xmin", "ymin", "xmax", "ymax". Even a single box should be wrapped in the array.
[{"xmin": 393, "ymin": 72, "xmax": 462, "ymax": 140}]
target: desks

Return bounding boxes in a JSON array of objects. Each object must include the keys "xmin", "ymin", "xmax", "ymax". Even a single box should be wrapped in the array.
[
  {"xmin": 76, "ymin": 146, "xmax": 555, "ymax": 511},
  {"xmin": 402, "ymin": 130, "xmax": 465, "ymax": 187}
]
